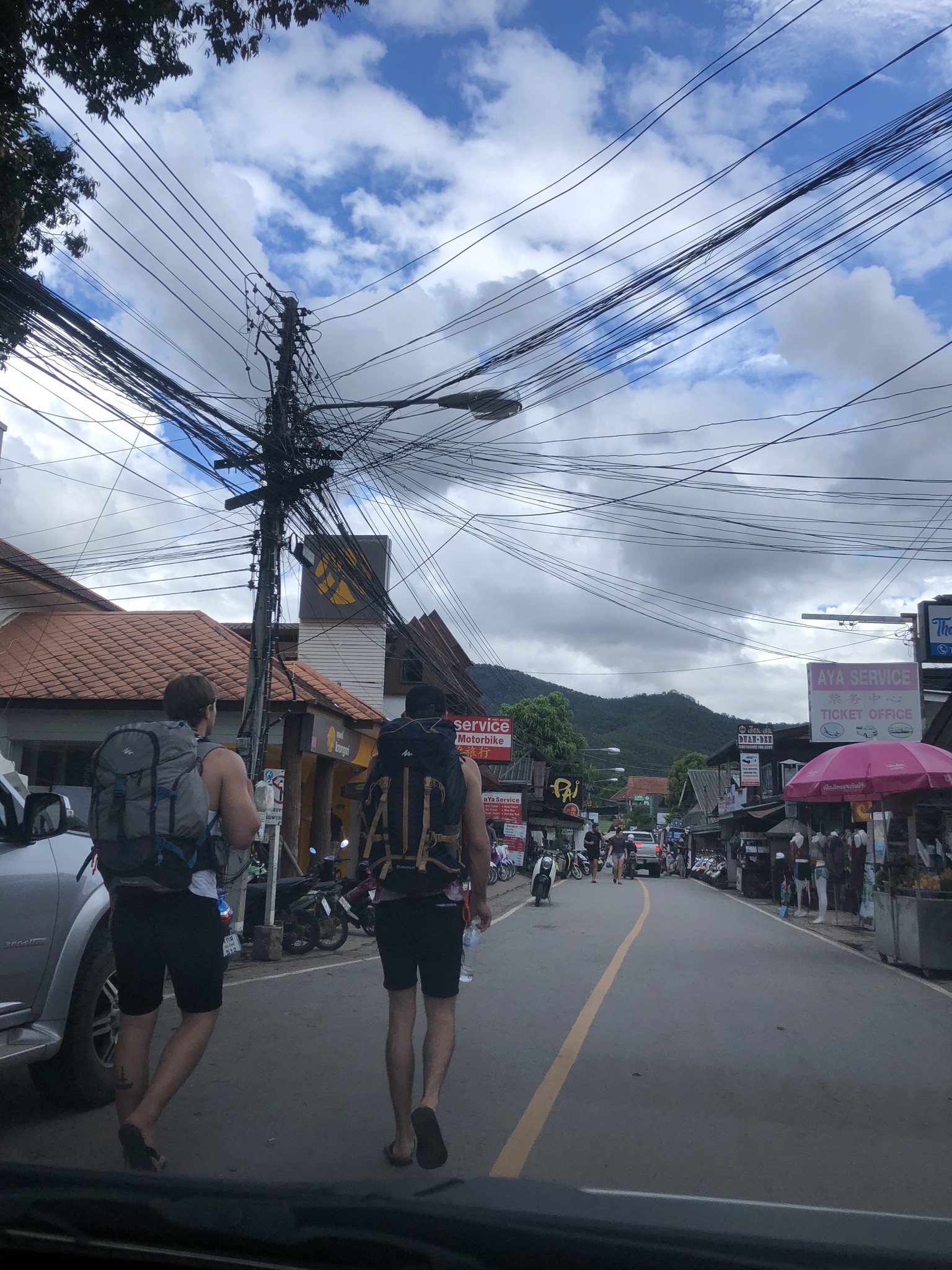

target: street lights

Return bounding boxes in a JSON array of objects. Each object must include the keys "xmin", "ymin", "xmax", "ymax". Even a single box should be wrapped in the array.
[{"xmin": 224, "ymin": 384, "xmax": 525, "ymax": 958}]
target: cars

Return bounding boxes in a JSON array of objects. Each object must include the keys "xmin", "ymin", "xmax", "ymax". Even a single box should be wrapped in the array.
[{"xmin": 0, "ymin": 773, "xmax": 121, "ymax": 1111}]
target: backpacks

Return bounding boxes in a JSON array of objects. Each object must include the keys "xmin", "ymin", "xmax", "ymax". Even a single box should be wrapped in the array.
[
  {"xmin": 87, "ymin": 721, "xmax": 226, "ymax": 891},
  {"xmin": 357, "ymin": 717, "xmax": 469, "ymax": 891}
]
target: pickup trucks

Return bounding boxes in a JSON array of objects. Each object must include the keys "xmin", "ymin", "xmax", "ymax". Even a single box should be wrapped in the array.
[{"xmin": 621, "ymin": 831, "xmax": 661, "ymax": 878}]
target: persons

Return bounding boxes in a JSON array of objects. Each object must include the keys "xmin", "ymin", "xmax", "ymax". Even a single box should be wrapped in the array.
[
  {"xmin": 625, "ymin": 834, "xmax": 638, "ymax": 859},
  {"xmin": 112, "ymin": 677, "xmax": 260, "ymax": 1172},
  {"xmin": 484, "ymin": 816, "xmax": 497, "ymax": 854},
  {"xmin": 660, "ymin": 839, "xmax": 671, "ymax": 869},
  {"xmin": 663, "ymin": 842, "xmax": 679, "ymax": 876},
  {"xmin": 790, "ymin": 828, "xmax": 868, "ymax": 925},
  {"xmin": 584, "ymin": 823, "xmax": 605, "ymax": 883},
  {"xmin": 330, "ymin": 807, "xmax": 345, "ymax": 856},
  {"xmin": 677, "ymin": 839, "xmax": 688, "ymax": 879},
  {"xmin": 374, "ymin": 687, "xmax": 494, "ymax": 1169},
  {"xmin": 608, "ymin": 827, "xmax": 627, "ymax": 884},
  {"xmin": 774, "ymin": 852, "xmax": 786, "ymax": 910}
]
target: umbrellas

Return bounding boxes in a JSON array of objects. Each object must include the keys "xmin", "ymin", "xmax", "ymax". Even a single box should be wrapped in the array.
[{"xmin": 783, "ymin": 736, "xmax": 952, "ymax": 967}]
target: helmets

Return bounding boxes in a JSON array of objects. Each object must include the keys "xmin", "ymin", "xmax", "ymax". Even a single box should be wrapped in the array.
[{"xmin": 627, "ymin": 834, "xmax": 634, "ymax": 841}]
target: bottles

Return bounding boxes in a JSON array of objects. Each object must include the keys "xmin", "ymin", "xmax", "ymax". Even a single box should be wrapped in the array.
[{"xmin": 459, "ymin": 920, "xmax": 480, "ymax": 982}]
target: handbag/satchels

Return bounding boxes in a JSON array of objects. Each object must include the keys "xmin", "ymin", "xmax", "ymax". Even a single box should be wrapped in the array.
[
  {"xmin": 675, "ymin": 855, "xmax": 679, "ymax": 861},
  {"xmin": 584, "ymin": 831, "xmax": 594, "ymax": 850},
  {"xmin": 606, "ymin": 856, "xmax": 614, "ymax": 868}
]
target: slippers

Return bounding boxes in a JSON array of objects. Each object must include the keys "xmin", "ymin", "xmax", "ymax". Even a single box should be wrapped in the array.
[
  {"xmin": 117, "ymin": 1123, "xmax": 168, "ymax": 1176},
  {"xmin": 410, "ymin": 1106, "xmax": 448, "ymax": 1170},
  {"xmin": 385, "ymin": 1138, "xmax": 416, "ymax": 1166}
]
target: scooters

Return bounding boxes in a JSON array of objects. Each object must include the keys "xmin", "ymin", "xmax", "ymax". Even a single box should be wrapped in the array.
[
  {"xmin": 623, "ymin": 852, "xmax": 636, "ymax": 879},
  {"xmin": 244, "ymin": 839, "xmax": 377, "ymax": 956},
  {"xmin": 487, "ymin": 841, "xmax": 606, "ymax": 907},
  {"xmin": 217, "ymin": 891, "xmax": 242, "ymax": 973},
  {"xmin": 690, "ymin": 848, "xmax": 728, "ymax": 890}
]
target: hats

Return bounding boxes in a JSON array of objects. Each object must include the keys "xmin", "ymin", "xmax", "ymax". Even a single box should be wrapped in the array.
[
  {"xmin": 405, "ymin": 684, "xmax": 445, "ymax": 719},
  {"xmin": 678, "ymin": 838, "xmax": 684, "ymax": 843}
]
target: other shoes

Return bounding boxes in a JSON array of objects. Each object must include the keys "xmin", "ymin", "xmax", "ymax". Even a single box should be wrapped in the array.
[
  {"xmin": 613, "ymin": 879, "xmax": 616, "ymax": 883},
  {"xmin": 618, "ymin": 882, "xmax": 622, "ymax": 884},
  {"xmin": 591, "ymin": 880, "xmax": 596, "ymax": 883},
  {"xmin": 680, "ymin": 877, "xmax": 687, "ymax": 879}
]
